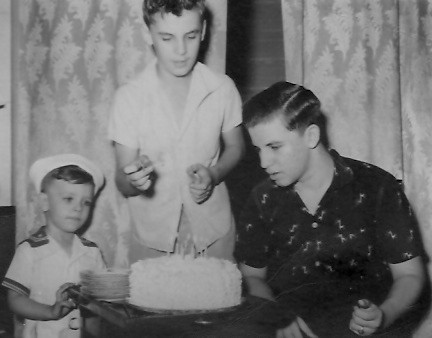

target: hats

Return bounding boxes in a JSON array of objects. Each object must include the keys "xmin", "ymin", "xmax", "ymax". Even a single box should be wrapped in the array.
[{"xmin": 29, "ymin": 154, "xmax": 106, "ymax": 197}]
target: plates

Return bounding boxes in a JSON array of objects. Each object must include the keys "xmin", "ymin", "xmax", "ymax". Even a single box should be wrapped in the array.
[{"xmin": 79, "ymin": 269, "xmax": 129, "ymax": 303}]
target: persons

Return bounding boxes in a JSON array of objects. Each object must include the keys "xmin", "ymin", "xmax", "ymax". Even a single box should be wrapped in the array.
[
  {"xmin": 3, "ymin": 153, "xmax": 110, "ymax": 338},
  {"xmin": 104, "ymin": 0, "xmax": 252, "ymax": 272},
  {"xmin": 231, "ymin": 80, "xmax": 429, "ymax": 337}
]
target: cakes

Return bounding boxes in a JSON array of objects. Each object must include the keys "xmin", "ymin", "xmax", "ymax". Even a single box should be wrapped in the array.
[{"xmin": 130, "ymin": 253, "xmax": 242, "ymax": 311}]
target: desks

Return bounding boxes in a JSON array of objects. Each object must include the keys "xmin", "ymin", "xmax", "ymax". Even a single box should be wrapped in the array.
[{"xmin": 67, "ymin": 285, "xmax": 284, "ymax": 338}]
